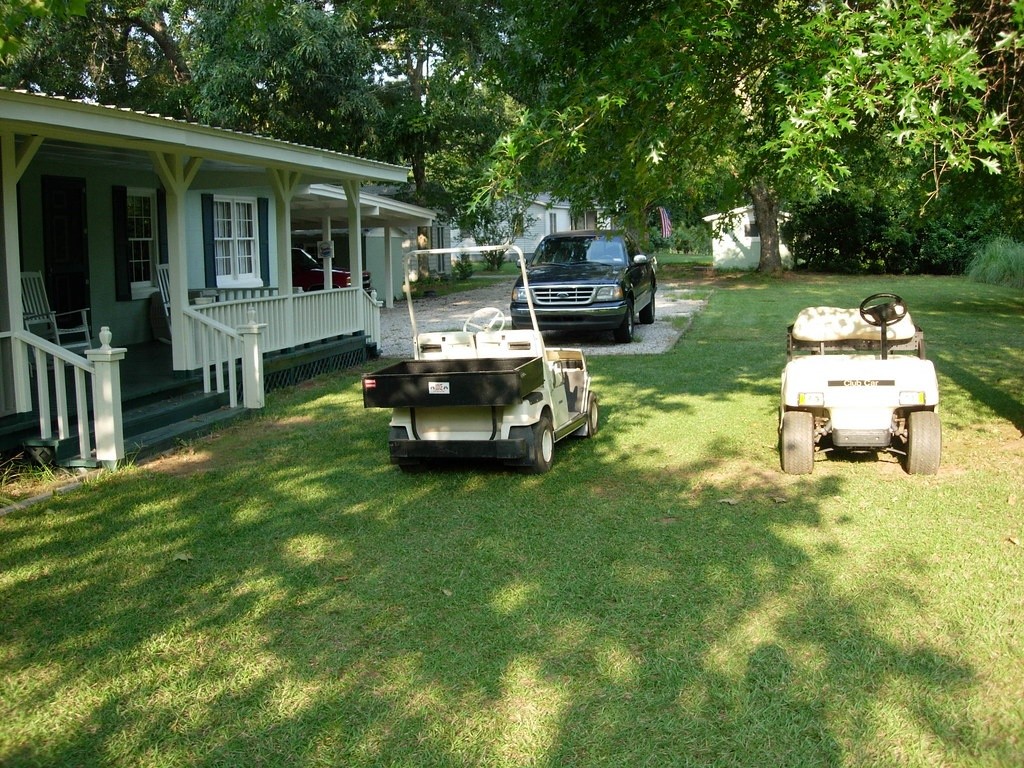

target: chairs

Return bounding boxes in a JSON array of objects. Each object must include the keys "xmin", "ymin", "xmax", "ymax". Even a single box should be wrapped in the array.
[
  {"xmin": 155, "ymin": 263, "xmax": 172, "ymax": 344},
  {"xmin": 417, "ymin": 331, "xmax": 477, "ymax": 359},
  {"xmin": 20, "ymin": 271, "xmax": 92, "ymax": 364},
  {"xmin": 475, "ymin": 329, "xmax": 543, "ymax": 357}
]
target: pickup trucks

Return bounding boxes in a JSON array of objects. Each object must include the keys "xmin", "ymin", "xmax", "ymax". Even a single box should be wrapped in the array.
[{"xmin": 509, "ymin": 229, "xmax": 658, "ymax": 344}]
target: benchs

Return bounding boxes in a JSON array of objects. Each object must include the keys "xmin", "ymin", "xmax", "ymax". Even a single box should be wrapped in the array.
[{"xmin": 792, "ymin": 305, "xmax": 916, "ymax": 356}]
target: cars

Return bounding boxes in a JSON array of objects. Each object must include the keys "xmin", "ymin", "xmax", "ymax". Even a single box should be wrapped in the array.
[{"xmin": 291, "ymin": 248, "xmax": 377, "ymax": 296}]
